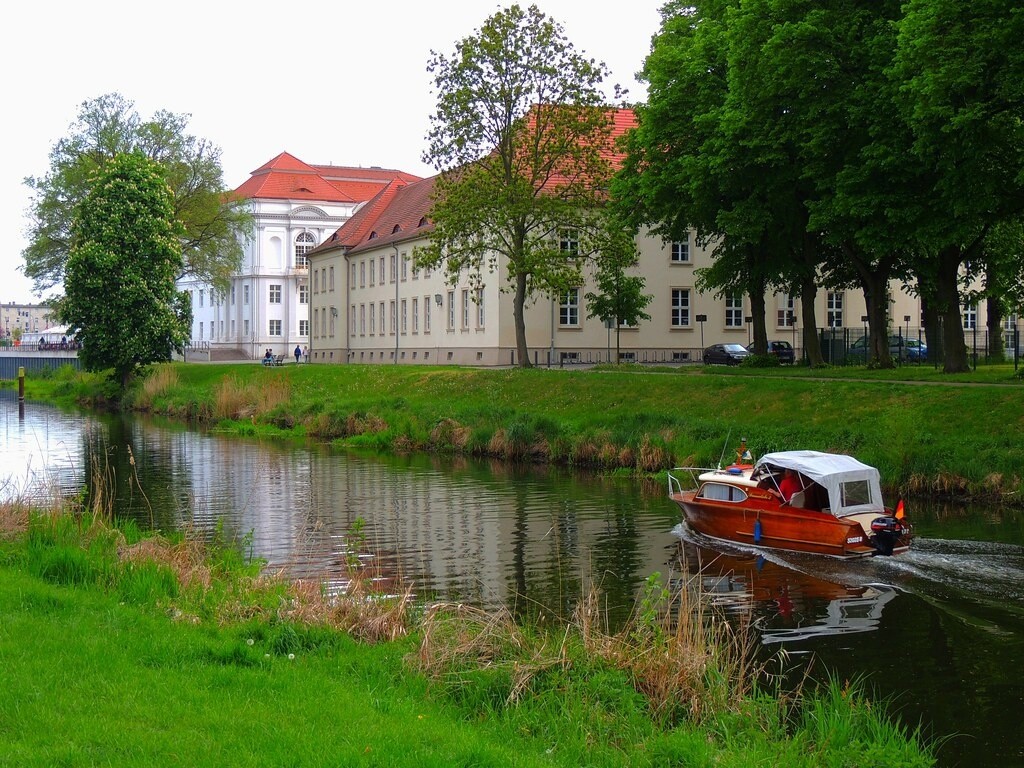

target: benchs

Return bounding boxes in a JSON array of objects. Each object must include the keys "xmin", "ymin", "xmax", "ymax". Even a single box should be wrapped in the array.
[
  {"xmin": 264, "ymin": 355, "xmax": 277, "ymax": 366},
  {"xmin": 619, "ymin": 352, "xmax": 635, "ymax": 363},
  {"xmin": 560, "ymin": 351, "xmax": 580, "ymax": 363},
  {"xmin": 672, "ymin": 353, "xmax": 690, "ymax": 362},
  {"xmin": 275, "ymin": 355, "xmax": 285, "ymax": 366}
]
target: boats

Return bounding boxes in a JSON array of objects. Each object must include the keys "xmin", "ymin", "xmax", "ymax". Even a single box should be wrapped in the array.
[
  {"xmin": 679, "ymin": 519, "xmax": 899, "ymax": 643},
  {"xmin": 666, "ymin": 429, "xmax": 914, "ymax": 562}
]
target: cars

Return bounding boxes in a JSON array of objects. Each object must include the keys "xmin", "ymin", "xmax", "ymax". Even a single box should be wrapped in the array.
[
  {"xmin": 903, "ymin": 337, "xmax": 928, "ymax": 362},
  {"xmin": 703, "ymin": 343, "xmax": 750, "ymax": 366}
]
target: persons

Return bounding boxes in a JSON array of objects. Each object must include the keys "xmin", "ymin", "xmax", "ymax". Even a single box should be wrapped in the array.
[
  {"xmin": 302, "ymin": 345, "xmax": 309, "ymax": 363},
  {"xmin": 293, "ymin": 344, "xmax": 301, "ymax": 363},
  {"xmin": 61, "ymin": 335, "xmax": 67, "ymax": 343},
  {"xmin": 263, "ymin": 349, "xmax": 273, "ymax": 358},
  {"xmin": 262, "ymin": 348, "xmax": 272, "ymax": 365},
  {"xmin": 39, "ymin": 336, "xmax": 46, "ymax": 349},
  {"xmin": 766, "ymin": 466, "xmax": 803, "ymax": 506}
]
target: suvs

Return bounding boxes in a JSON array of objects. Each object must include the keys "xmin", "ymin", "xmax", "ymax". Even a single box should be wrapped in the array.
[
  {"xmin": 848, "ymin": 333, "xmax": 906, "ymax": 362},
  {"xmin": 745, "ymin": 340, "xmax": 796, "ymax": 366}
]
